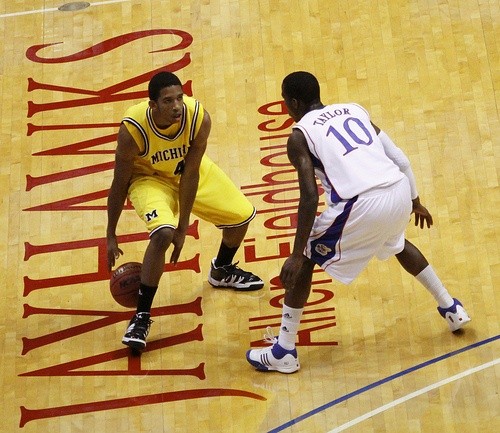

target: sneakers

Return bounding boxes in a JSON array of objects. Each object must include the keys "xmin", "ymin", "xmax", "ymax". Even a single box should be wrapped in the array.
[
  {"xmin": 121, "ymin": 315, "xmax": 150, "ymax": 353},
  {"xmin": 437, "ymin": 297, "xmax": 471, "ymax": 332},
  {"xmin": 245, "ymin": 342, "xmax": 300, "ymax": 374},
  {"xmin": 209, "ymin": 259, "xmax": 264, "ymax": 291}
]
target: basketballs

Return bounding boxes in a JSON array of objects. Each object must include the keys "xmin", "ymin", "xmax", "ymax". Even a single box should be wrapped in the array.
[{"xmin": 110, "ymin": 263, "xmax": 141, "ymax": 308}]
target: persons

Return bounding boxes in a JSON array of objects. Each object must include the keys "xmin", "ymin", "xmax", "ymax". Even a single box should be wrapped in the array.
[
  {"xmin": 106, "ymin": 70, "xmax": 265, "ymax": 350},
  {"xmin": 245, "ymin": 71, "xmax": 470, "ymax": 375}
]
